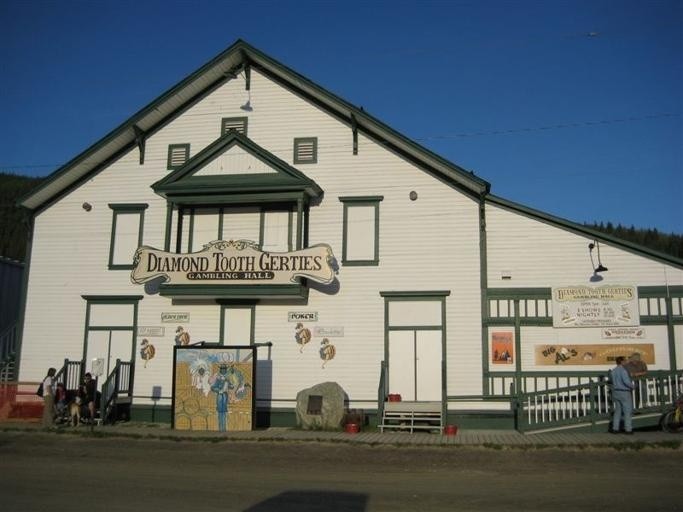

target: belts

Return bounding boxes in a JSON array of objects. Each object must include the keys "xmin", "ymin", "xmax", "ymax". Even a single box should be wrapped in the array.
[{"xmin": 614, "ymin": 389, "xmax": 629, "ymax": 391}]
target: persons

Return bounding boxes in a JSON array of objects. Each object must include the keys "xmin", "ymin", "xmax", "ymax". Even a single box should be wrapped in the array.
[
  {"xmin": 175, "ymin": 327, "xmax": 188, "ymax": 346},
  {"xmin": 53, "ymin": 382, "xmax": 68, "ymax": 423},
  {"xmin": 319, "ymin": 339, "xmax": 334, "ymax": 369},
  {"xmin": 42, "ymin": 368, "xmax": 58, "ymax": 430},
  {"xmin": 76, "ymin": 372, "xmax": 98, "ymax": 421},
  {"xmin": 140, "ymin": 339, "xmax": 153, "ymax": 370},
  {"xmin": 209, "ymin": 363, "xmax": 233, "ymax": 432},
  {"xmin": 295, "ymin": 324, "xmax": 308, "ymax": 354},
  {"xmin": 624, "ymin": 352, "xmax": 648, "ymax": 410},
  {"xmin": 607, "ymin": 355, "xmax": 639, "ymax": 434}
]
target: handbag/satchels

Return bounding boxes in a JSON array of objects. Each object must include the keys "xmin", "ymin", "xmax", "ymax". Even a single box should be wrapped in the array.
[{"xmin": 36, "ymin": 375, "xmax": 48, "ymax": 397}]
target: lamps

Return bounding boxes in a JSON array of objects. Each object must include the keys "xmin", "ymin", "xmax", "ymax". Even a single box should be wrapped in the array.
[
  {"xmin": 224, "ymin": 64, "xmax": 248, "ymax": 80},
  {"xmin": 589, "ymin": 238, "xmax": 608, "ymax": 271}
]
